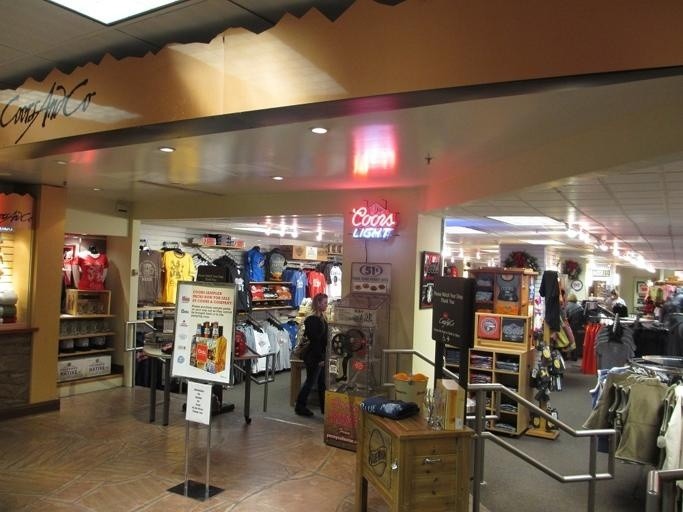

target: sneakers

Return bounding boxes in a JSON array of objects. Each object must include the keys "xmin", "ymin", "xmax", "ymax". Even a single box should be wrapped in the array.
[{"xmin": 294, "ymin": 407, "xmax": 315, "ymax": 415}]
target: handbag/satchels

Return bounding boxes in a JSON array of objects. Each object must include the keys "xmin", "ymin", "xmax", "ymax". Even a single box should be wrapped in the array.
[{"xmin": 293, "ymin": 335, "xmax": 310, "ymax": 357}]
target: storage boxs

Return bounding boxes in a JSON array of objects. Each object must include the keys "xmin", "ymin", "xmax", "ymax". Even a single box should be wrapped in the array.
[
  {"xmin": 58, "ymin": 355, "xmax": 112, "ymax": 382},
  {"xmin": 282, "ymin": 245, "xmax": 328, "ymax": 260}
]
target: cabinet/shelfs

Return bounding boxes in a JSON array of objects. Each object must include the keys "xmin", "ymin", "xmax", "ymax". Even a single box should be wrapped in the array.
[
  {"xmin": 467, "ymin": 312, "xmax": 534, "ymax": 436},
  {"xmin": 58, "ymin": 315, "xmax": 116, "ymax": 357},
  {"xmin": 355, "ymin": 407, "xmax": 475, "ymax": 512},
  {"xmin": 248, "ymin": 281, "xmax": 293, "ymax": 310}
]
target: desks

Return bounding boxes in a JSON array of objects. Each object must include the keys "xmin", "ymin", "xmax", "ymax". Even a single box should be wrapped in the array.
[{"xmin": 143, "ymin": 344, "xmax": 257, "ymax": 426}]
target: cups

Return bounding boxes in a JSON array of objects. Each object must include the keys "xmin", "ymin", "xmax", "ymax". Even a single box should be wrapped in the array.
[{"xmin": 426, "ymin": 386, "xmax": 447, "ymax": 432}]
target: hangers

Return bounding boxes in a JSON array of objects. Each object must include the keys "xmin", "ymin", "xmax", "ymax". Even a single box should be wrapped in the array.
[
  {"xmin": 245, "ymin": 310, "xmax": 298, "ymax": 333},
  {"xmin": 192, "ymin": 247, "xmax": 240, "ymax": 269},
  {"xmin": 286, "ymin": 256, "xmax": 339, "ymax": 272},
  {"xmin": 625, "ymin": 358, "xmax": 683, "ymax": 386},
  {"xmin": 569, "ymin": 304, "xmax": 671, "ymax": 345},
  {"xmin": 140, "ymin": 239, "xmax": 184, "ymax": 255}
]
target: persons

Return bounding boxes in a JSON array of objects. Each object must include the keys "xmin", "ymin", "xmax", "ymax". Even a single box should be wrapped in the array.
[
  {"xmin": 563, "ymin": 294, "xmax": 586, "ymax": 361},
  {"xmin": 245, "ymin": 246, "xmax": 266, "ymax": 282},
  {"xmin": 605, "ymin": 289, "xmax": 626, "ymax": 311},
  {"xmin": 294, "ymin": 293, "xmax": 329, "ymax": 417},
  {"xmin": 78, "ymin": 243, "xmax": 108, "ymax": 290},
  {"xmin": 265, "ymin": 248, "xmax": 287, "ymax": 281}
]
